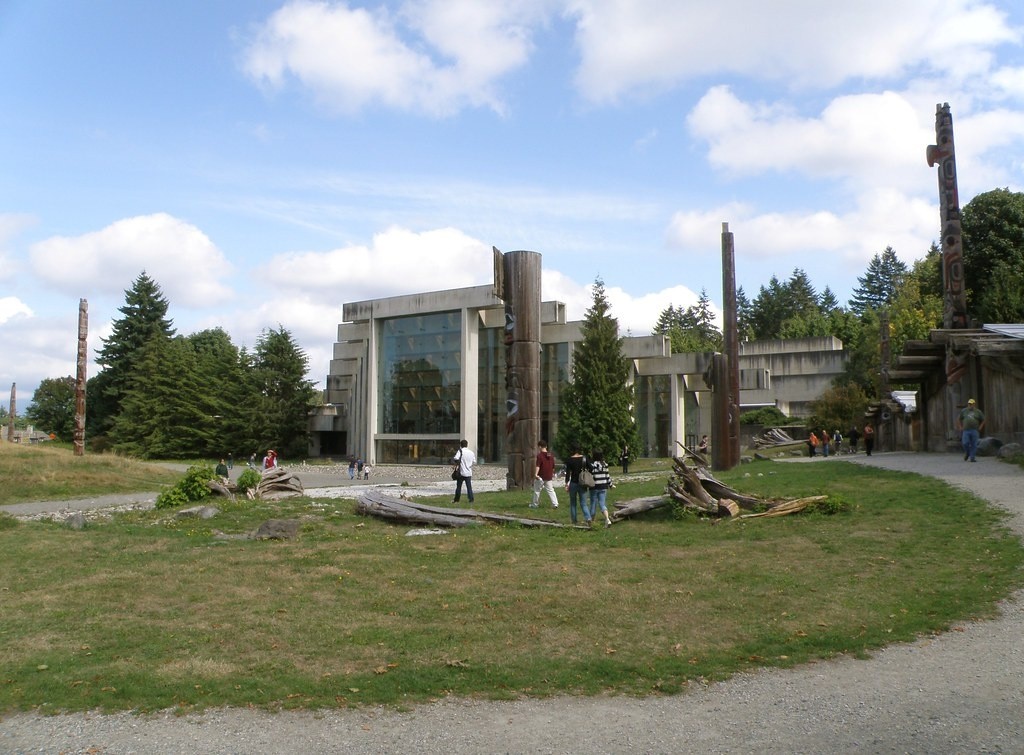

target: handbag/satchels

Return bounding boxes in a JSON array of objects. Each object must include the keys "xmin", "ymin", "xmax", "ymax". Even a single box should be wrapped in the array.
[
  {"xmin": 578, "ymin": 454, "xmax": 595, "ymax": 488},
  {"xmin": 452, "ymin": 469, "xmax": 462, "ymax": 480}
]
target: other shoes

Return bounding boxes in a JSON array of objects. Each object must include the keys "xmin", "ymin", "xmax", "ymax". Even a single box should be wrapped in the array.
[
  {"xmin": 964, "ymin": 455, "xmax": 969, "ymax": 461},
  {"xmin": 452, "ymin": 501, "xmax": 459, "ymax": 505},
  {"xmin": 605, "ymin": 519, "xmax": 611, "ymax": 528}
]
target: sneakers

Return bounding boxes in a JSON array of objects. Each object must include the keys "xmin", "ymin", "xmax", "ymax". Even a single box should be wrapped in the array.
[{"xmin": 528, "ymin": 503, "xmax": 540, "ymax": 508}]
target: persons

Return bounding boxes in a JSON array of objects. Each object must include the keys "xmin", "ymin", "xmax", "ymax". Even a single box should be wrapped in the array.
[
  {"xmin": 564, "ymin": 441, "xmax": 593, "ymax": 527},
  {"xmin": 349, "ymin": 455, "xmax": 370, "ymax": 481},
  {"xmin": 958, "ymin": 398, "xmax": 987, "ymax": 462},
  {"xmin": 263, "ymin": 449, "xmax": 278, "ymax": 471},
  {"xmin": 848, "ymin": 427, "xmax": 861, "ymax": 455},
  {"xmin": 864, "ymin": 422, "xmax": 874, "ymax": 457},
  {"xmin": 228, "ymin": 452, "xmax": 233, "ymax": 470},
  {"xmin": 805, "ymin": 429, "xmax": 844, "ymax": 458},
  {"xmin": 249, "ymin": 452, "xmax": 257, "ymax": 471},
  {"xmin": 529, "ymin": 439, "xmax": 561, "ymax": 509},
  {"xmin": 698, "ymin": 435, "xmax": 709, "ymax": 469},
  {"xmin": 619, "ymin": 445, "xmax": 631, "ymax": 474},
  {"xmin": 452, "ymin": 440, "xmax": 476, "ymax": 504},
  {"xmin": 588, "ymin": 447, "xmax": 617, "ymax": 529},
  {"xmin": 215, "ymin": 457, "xmax": 229, "ymax": 486}
]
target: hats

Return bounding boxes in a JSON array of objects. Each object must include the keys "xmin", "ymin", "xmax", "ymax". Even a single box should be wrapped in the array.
[{"xmin": 967, "ymin": 399, "xmax": 976, "ymax": 404}]
